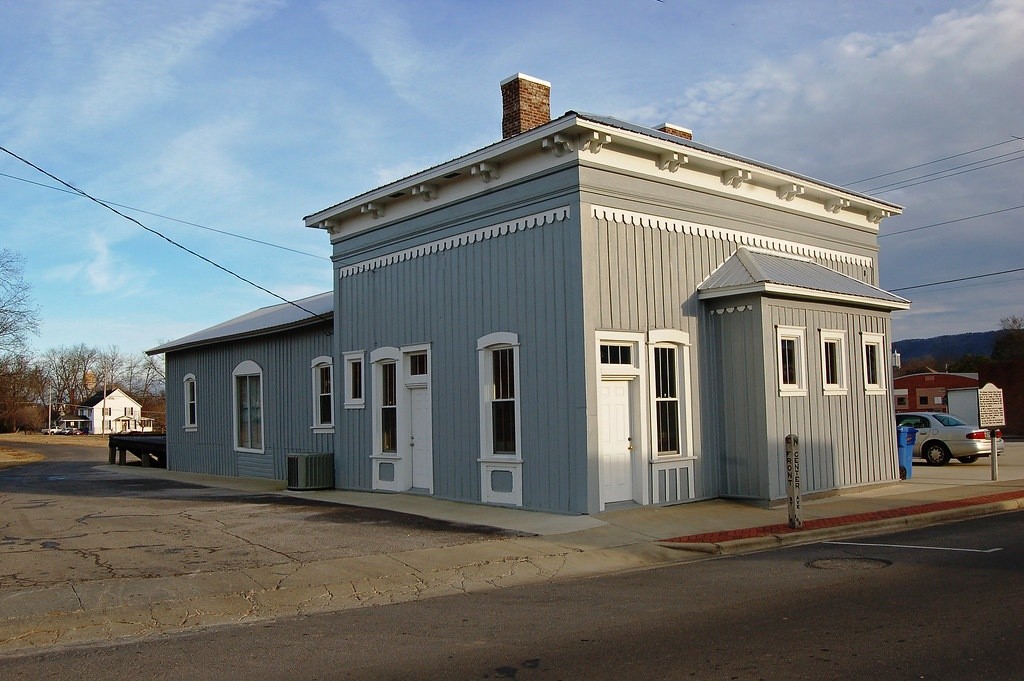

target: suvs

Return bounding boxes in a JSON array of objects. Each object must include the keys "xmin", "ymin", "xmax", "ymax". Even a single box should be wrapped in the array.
[{"xmin": 61, "ymin": 427, "xmax": 77, "ymax": 434}]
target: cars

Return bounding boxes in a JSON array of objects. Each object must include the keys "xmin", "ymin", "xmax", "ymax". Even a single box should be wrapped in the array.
[{"xmin": 897, "ymin": 411, "xmax": 1006, "ymax": 466}]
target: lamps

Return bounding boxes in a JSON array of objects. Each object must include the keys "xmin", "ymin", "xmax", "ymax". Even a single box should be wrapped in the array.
[{"xmin": 892, "ymin": 349, "xmax": 901, "ymax": 369}]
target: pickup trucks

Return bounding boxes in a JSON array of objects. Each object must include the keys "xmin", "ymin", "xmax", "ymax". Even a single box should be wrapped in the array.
[{"xmin": 42, "ymin": 426, "xmax": 62, "ymax": 435}]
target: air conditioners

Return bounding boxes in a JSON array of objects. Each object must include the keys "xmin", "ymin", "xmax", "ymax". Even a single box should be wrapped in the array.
[{"xmin": 286, "ymin": 452, "xmax": 336, "ymax": 490}]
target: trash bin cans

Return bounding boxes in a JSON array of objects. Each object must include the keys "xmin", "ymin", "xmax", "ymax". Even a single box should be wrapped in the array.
[{"xmin": 896, "ymin": 424, "xmax": 920, "ymax": 482}]
